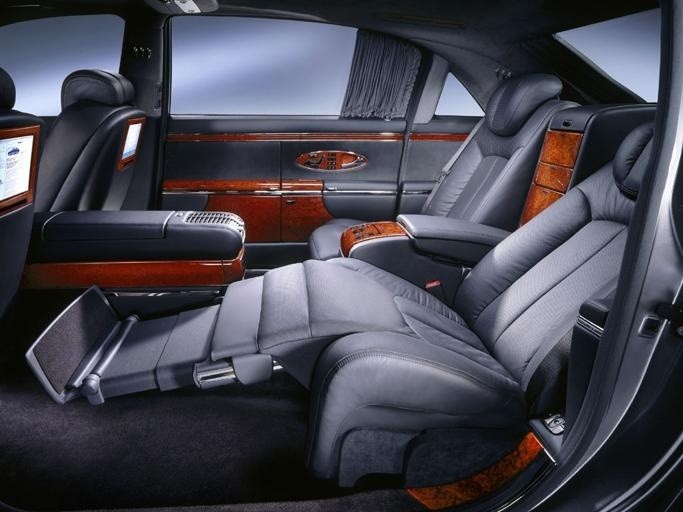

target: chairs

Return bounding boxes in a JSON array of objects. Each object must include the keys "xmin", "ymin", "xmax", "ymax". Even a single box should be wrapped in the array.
[{"xmin": 2, "ymin": 71, "xmax": 660, "ymax": 512}]
